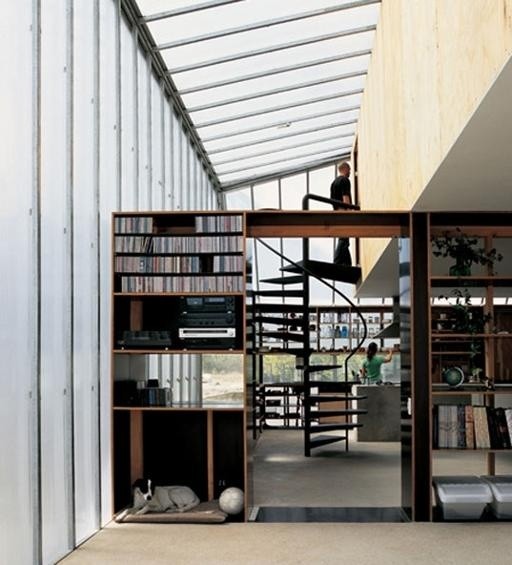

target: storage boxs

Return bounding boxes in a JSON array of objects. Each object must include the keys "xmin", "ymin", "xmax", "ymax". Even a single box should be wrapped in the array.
[{"xmin": 431, "ymin": 472, "xmax": 512, "ymax": 520}]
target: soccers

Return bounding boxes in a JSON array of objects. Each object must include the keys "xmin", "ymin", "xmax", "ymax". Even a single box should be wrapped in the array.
[{"xmin": 219, "ymin": 487, "xmax": 244, "ymax": 513}]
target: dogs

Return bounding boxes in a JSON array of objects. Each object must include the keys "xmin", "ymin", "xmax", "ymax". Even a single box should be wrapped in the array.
[{"xmin": 115, "ymin": 478, "xmax": 200, "ymax": 522}]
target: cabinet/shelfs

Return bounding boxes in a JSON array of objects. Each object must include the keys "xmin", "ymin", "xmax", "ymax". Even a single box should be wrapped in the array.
[
  {"xmin": 110, "ymin": 210, "xmax": 247, "ymax": 522},
  {"xmin": 431, "ymin": 225, "xmax": 512, "ymax": 520},
  {"xmin": 308, "ymin": 309, "xmax": 399, "ymax": 352}
]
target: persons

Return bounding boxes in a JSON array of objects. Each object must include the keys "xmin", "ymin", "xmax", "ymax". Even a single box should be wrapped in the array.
[
  {"xmin": 331, "ymin": 163, "xmax": 351, "ymax": 266},
  {"xmin": 360, "ymin": 343, "xmax": 392, "ymax": 385}
]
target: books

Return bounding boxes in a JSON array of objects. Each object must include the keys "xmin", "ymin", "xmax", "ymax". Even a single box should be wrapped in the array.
[
  {"xmin": 433, "ymin": 405, "xmax": 512, "ymax": 449},
  {"xmin": 114, "ymin": 216, "xmax": 244, "ymax": 293}
]
